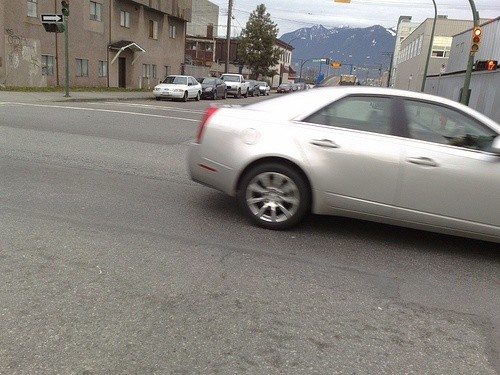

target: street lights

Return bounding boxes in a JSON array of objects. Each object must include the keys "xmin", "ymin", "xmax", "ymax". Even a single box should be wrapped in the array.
[{"xmin": 281, "ymin": 37, "xmax": 306, "ymax": 83}]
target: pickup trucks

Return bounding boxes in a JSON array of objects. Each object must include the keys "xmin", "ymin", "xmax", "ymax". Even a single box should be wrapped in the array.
[{"xmin": 218, "ymin": 74, "xmax": 250, "ymax": 99}]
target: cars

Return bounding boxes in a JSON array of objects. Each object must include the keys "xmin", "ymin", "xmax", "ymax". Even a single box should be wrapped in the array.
[
  {"xmin": 185, "ymin": 83, "xmax": 500, "ymax": 247},
  {"xmin": 245, "ymin": 78, "xmax": 271, "ymax": 97},
  {"xmin": 196, "ymin": 76, "xmax": 228, "ymax": 100},
  {"xmin": 277, "ymin": 83, "xmax": 316, "ymax": 93},
  {"xmin": 152, "ymin": 74, "xmax": 202, "ymax": 102}
]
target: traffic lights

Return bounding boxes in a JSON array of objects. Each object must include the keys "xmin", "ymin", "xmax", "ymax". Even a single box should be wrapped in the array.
[
  {"xmin": 470, "ymin": 26, "xmax": 483, "ymax": 52},
  {"xmin": 474, "ymin": 59, "xmax": 498, "ymax": 70}
]
